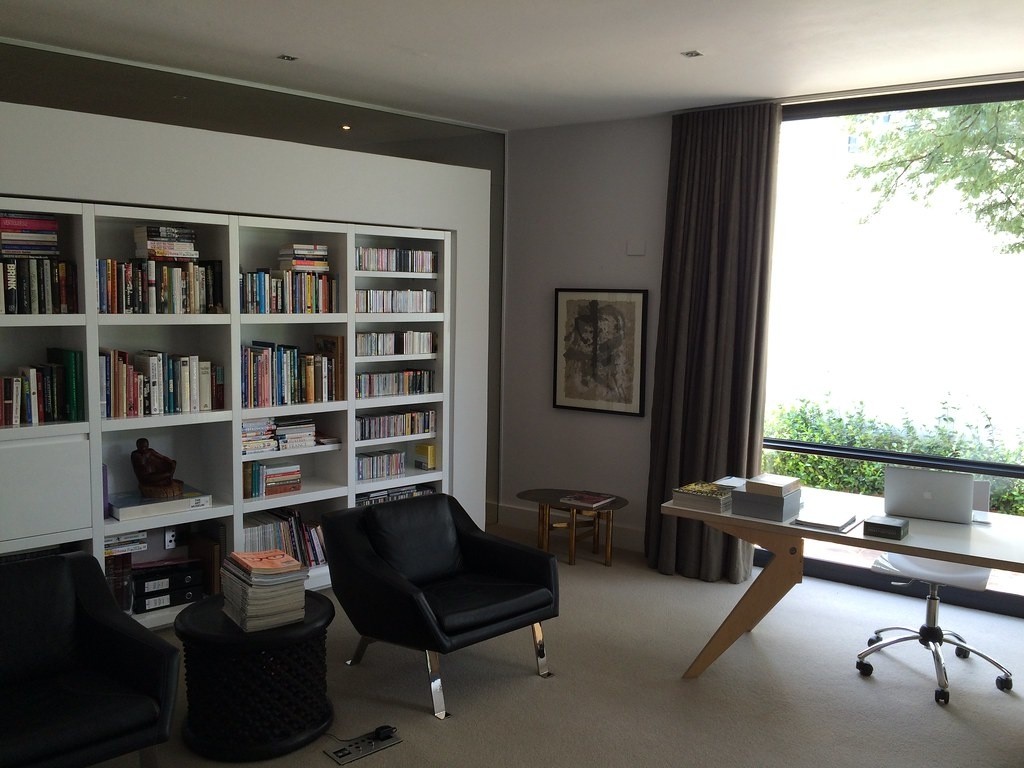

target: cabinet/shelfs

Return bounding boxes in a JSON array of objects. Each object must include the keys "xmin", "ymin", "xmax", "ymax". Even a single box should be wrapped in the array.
[{"xmin": 0, "ymin": 197, "xmax": 452, "ymax": 631}]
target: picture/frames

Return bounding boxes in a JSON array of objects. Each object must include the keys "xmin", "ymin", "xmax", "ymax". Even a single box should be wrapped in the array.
[{"xmin": 551, "ymin": 287, "xmax": 650, "ymax": 417}]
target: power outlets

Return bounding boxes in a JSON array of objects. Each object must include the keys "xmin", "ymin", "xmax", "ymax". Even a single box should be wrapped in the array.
[{"xmin": 324, "ymin": 729, "xmax": 405, "ymax": 766}]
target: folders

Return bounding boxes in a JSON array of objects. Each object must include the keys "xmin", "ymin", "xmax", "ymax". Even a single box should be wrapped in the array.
[{"xmin": 131, "ymin": 564, "xmax": 206, "ymax": 614}]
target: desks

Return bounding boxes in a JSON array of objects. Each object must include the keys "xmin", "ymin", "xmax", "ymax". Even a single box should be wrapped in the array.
[
  {"xmin": 660, "ymin": 484, "xmax": 1024, "ymax": 683},
  {"xmin": 174, "ymin": 589, "xmax": 335, "ymax": 763},
  {"xmin": 519, "ymin": 490, "xmax": 630, "ymax": 568}
]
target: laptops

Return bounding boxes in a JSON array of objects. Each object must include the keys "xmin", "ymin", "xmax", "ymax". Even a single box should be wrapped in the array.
[{"xmin": 884, "ymin": 466, "xmax": 975, "ymax": 523}]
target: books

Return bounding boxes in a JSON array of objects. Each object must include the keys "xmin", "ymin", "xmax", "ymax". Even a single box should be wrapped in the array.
[
  {"xmin": 0, "ymin": 211, "xmax": 439, "ymax": 634},
  {"xmin": 673, "ymin": 475, "xmax": 859, "ymax": 533},
  {"xmin": 559, "ymin": 492, "xmax": 616, "ymax": 508}
]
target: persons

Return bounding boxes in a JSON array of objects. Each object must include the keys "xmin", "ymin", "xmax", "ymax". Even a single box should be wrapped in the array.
[{"xmin": 131, "ymin": 438, "xmax": 176, "ymax": 486}]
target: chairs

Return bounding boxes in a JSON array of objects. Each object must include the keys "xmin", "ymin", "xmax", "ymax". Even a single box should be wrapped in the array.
[
  {"xmin": 852, "ymin": 478, "xmax": 1013, "ymax": 708},
  {"xmin": 0, "ymin": 551, "xmax": 181, "ymax": 768},
  {"xmin": 319, "ymin": 492, "xmax": 561, "ymax": 719}
]
